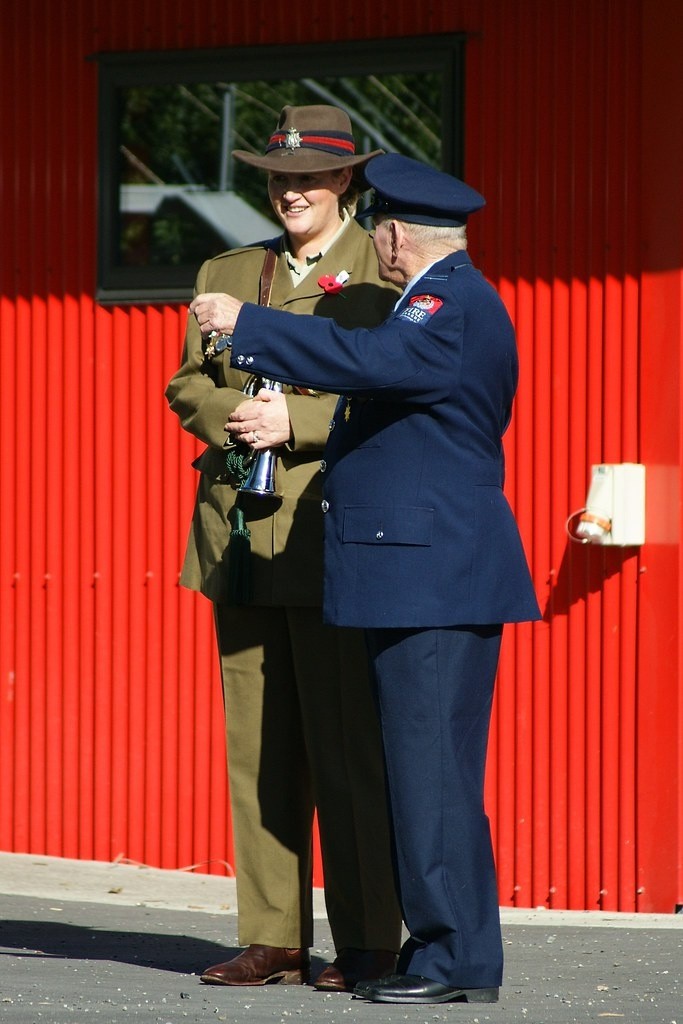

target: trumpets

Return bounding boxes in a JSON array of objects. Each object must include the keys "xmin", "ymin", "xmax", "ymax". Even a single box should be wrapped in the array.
[{"xmin": 234, "ymin": 374, "xmax": 285, "ymax": 504}]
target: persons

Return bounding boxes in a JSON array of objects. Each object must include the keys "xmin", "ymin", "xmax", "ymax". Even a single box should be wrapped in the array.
[
  {"xmin": 165, "ymin": 104, "xmax": 405, "ymax": 990},
  {"xmin": 185, "ymin": 154, "xmax": 543, "ymax": 1005}
]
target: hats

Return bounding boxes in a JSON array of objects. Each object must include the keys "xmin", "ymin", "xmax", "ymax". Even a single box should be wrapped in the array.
[
  {"xmin": 231, "ymin": 105, "xmax": 385, "ymax": 194},
  {"xmin": 353, "ymin": 152, "xmax": 486, "ymax": 227}
]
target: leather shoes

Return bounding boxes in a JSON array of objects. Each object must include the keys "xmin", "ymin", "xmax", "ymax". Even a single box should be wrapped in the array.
[
  {"xmin": 354, "ymin": 974, "xmax": 404, "ymax": 995},
  {"xmin": 364, "ymin": 976, "xmax": 498, "ymax": 1003},
  {"xmin": 314, "ymin": 964, "xmax": 352, "ymax": 990},
  {"xmin": 200, "ymin": 945, "xmax": 311, "ymax": 984}
]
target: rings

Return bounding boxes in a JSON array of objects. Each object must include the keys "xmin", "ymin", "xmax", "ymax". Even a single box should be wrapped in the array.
[{"xmin": 252, "ymin": 430, "xmax": 258, "ymax": 442}]
